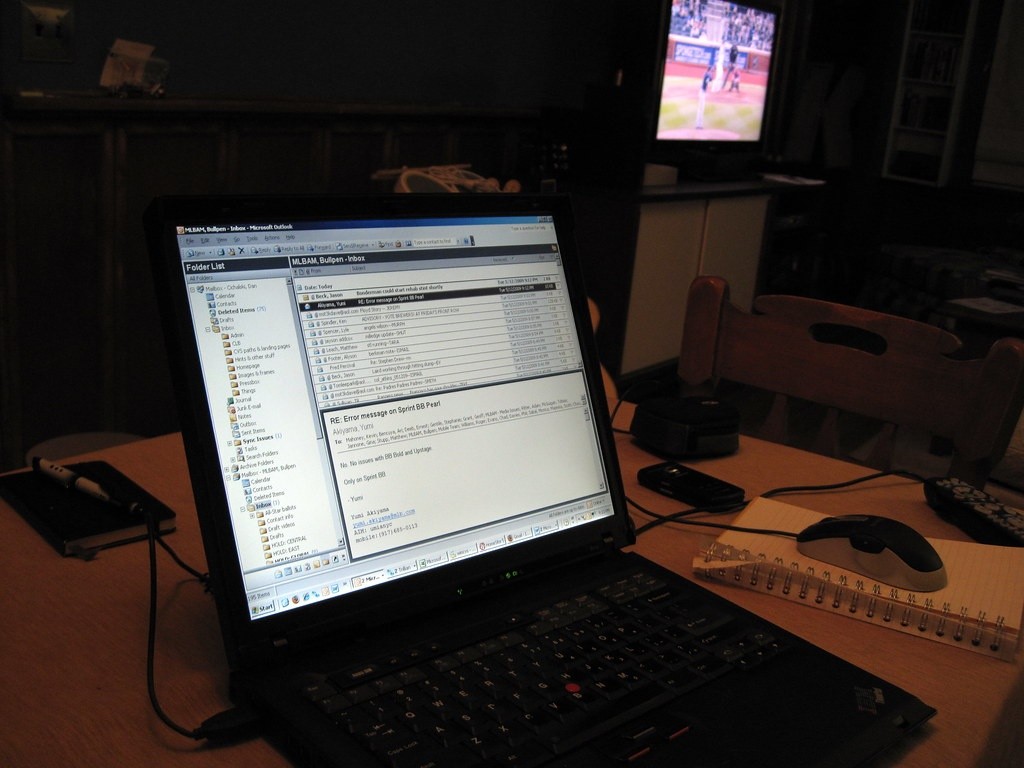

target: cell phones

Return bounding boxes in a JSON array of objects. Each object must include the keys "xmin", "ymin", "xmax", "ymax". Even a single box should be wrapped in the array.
[{"xmin": 638, "ymin": 460, "xmax": 744, "ymax": 513}]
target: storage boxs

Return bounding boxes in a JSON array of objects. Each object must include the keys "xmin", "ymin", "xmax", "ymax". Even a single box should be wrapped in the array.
[{"xmin": 99, "ymin": 37, "xmax": 171, "ymax": 90}]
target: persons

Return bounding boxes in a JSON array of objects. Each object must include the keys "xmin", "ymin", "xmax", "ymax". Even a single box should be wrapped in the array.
[
  {"xmin": 670, "ymin": 0, "xmax": 776, "ymax": 92},
  {"xmin": 695, "ymin": 66, "xmax": 713, "ymax": 129}
]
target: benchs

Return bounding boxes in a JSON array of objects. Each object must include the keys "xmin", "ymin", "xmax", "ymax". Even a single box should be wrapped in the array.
[{"xmin": 858, "ymin": 244, "xmax": 1024, "ymax": 346}]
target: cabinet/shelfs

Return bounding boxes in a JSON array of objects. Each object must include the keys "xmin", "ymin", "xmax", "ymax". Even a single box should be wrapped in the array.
[
  {"xmin": 571, "ymin": 170, "xmax": 828, "ymax": 386},
  {"xmin": 882, "ymin": 0, "xmax": 980, "ymax": 186}
]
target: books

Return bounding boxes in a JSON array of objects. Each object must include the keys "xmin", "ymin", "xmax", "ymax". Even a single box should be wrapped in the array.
[
  {"xmin": 693, "ymin": 496, "xmax": 1024, "ymax": 663},
  {"xmin": 0, "ymin": 461, "xmax": 177, "ymax": 558}
]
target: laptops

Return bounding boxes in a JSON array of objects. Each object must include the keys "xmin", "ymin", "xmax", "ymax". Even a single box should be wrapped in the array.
[{"xmin": 135, "ymin": 191, "xmax": 942, "ymax": 768}]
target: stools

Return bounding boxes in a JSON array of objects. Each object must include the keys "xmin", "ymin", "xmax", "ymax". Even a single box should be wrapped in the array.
[{"xmin": 27, "ymin": 429, "xmax": 148, "ymax": 467}]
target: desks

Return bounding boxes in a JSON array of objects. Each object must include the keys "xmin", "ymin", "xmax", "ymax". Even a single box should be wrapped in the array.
[{"xmin": 0, "ymin": 397, "xmax": 1024, "ymax": 768}]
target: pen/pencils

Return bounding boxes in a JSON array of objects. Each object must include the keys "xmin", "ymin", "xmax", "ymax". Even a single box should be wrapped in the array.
[{"xmin": 31, "ymin": 457, "xmax": 143, "ymax": 514}]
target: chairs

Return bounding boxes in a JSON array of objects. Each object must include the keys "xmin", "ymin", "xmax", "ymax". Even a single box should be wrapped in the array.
[{"xmin": 678, "ymin": 275, "xmax": 1024, "ymax": 491}]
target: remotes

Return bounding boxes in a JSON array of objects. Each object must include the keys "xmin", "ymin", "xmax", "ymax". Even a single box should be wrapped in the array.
[{"xmin": 922, "ymin": 477, "xmax": 1024, "ymax": 549}]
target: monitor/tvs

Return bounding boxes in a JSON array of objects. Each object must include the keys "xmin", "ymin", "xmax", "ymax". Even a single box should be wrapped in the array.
[{"xmin": 612, "ymin": 0, "xmax": 793, "ymax": 180}]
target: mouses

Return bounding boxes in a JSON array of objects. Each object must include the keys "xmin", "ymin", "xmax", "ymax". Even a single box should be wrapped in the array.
[{"xmin": 796, "ymin": 514, "xmax": 948, "ymax": 592}]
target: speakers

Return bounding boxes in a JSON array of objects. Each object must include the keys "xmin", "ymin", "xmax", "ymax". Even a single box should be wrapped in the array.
[{"xmin": 629, "ymin": 396, "xmax": 739, "ymax": 460}]
